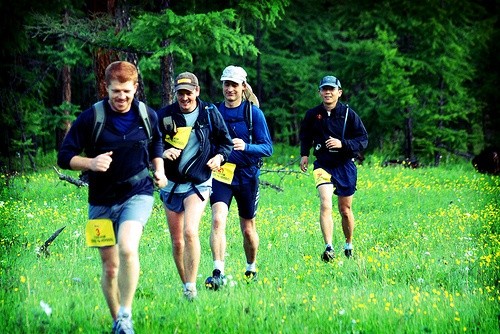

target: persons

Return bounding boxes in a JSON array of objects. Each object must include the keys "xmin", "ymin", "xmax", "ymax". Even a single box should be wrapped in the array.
[
  {"xmin": 300, "ymin": 77, "xmax": 369, "ymax": 263},
  {"xmin": 204, "ymin": 65, "xmax": 274, "ymax": 292},
  {"xmin": 150, "ymin": 72, "xmax": 235, "ymax": 302},
  {"xmin": 57, "ymin": 61, "xmax": 168, "ymax": 334}
]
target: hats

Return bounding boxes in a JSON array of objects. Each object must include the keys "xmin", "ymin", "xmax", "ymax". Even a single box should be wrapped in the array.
[
  {"xmin": 318, "ymin": 75, "xmax": 342, "ymax": 89},
  {"xmin": 221, "ymin": 66, "xmax": 247, "ymax": 84},
  {"xmin": 174, "ymin": 71, "xmax": 199, "ymax": 91}
]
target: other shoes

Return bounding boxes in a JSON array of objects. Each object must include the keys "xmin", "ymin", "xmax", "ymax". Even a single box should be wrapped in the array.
[
  {"xmin": 205, "ymin": 269, "xmax": 227, "ymax": 291},
  {"xmin": 345, "ymin": 248, "xmax": 352, "ymax": 260},
  {"xmin": 112, "ymin": 311, "xmax": 135, "ymax": 334},
  {"xmin": 244, "ymin": 271, "xmax": 257, "ymax": 284},
  {"xmin": 183, "ymin": 288, "xmax": 197, "ymax": 301},
  {"xmin": 320, "ymin": 246, "xmax": 335, "ymax": 262}
]
